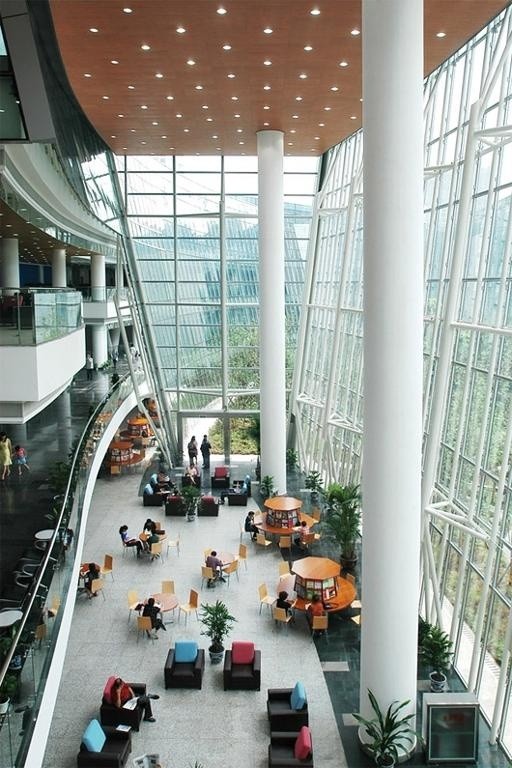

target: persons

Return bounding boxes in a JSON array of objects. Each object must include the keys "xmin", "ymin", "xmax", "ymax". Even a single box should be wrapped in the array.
[
  {"xmin": 81, "ymin": 563, "xmax": 99, "ymax": 596},
  {"xmin": 295, "ymin": 521, "xmax": 309, "ymax": 551},
  {"xmin": 276, "ymin": 591, "xmax": 297, "ymax": 616},
  {"xmin": 143, "ymin": 598, "xmax": 162, "ymax": 639},
  {"xmin": 188, "ymin": 434, "xmax": 211, "ymax": 468},
  {"xmin": 111, "ymin": 678, "xmax": 159, "ymax": 722},
  {"xmin": 112, "ymin": 349, "xmax": 119, "ymax": 368},
  {"xmin": 309, "ymin": 595, "xmax": 327, "ymax": 637},
  {"xmin": 245, "ymin": 511, "xmax": 259, "ymax": 540},
  {"xmin": 154, "ymin": 472, "xmax": 176, "ymax": 500},
  {"xmin": 206, "ymin": 550, "xmax": 223, "ymax": 588},
  {"xmin": 86, "ymin": 353, "xmax": 93, "ymax": 380},
  {"xmin": 187, "ymin": 464, "xmax": 197, "ymax": 485},
  {"xmin": 218, "ymin": 484, "xmax": 243, "ymax": 505},
  {"xmin": 1, "ymin": 432, "xmax": 29, "ymax": 480},
  {"xmin": 120, "ymin": 518, "xmax": 159, "ymax": 554},
  {"xmin": 130, "ymin": 345, "xmax": 136, "ymax": 362},
  {"xmin": 112, "ymin": 374, "xmax": 119, "ymax": 389}
]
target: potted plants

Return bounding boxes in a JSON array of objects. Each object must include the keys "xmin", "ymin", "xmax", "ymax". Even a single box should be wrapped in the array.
[
  {"xmin": 175, "ymin": 484, "xmax": 204, "ymax": 520},
  {"xmin": 324, "ymin": 481, "xmax": 362, "ymax": 569},
  {"xmin": 199, "ymin": 598, "xmax": 239, "ymax": 661},
  {"xmin": 286, "ymin": 449, "xmax": 300, "ymax": 469},
  {"xmin": 352, "ymin": 687, "xmax": 429, "ymax": 768},
  {"xmin": 304, "ymin": 469, "xmax": 324, "ymax": 501},
  {"xmin": 2, "ymin": 622, "xmax": 28, "ymax": 669},
  {"xmin": 419, "ymin": 625, "xmax": 456, "ymax": 691},
  {"xmin": 259, "ymin": 475, "xmax": 276, "ymax": 502},
  {"xmin": 0, "ymin": 673, "xmax": 18, "ymax": 714}
]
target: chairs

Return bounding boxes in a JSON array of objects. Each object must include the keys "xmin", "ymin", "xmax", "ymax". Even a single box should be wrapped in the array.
[
  {"xmin": 1, "ymin": 527, "xmax": 66, "ymax": 625},
  {"xmin": 77, "ymin": 718, "xmax": 132, "ymax": 767},
  {"xmin": 102, "ymin": 678, "xmax": 147, "ymax": 732},
  {"xmin": 257, "ymin": 558, "xmax": 361, "ymax": 644},
  {"xmin": 164, "ymin": 640, "xmax": 205, "ymax": 690},
  {"xmin": 223, "ymin": 639, "xmax": 261, "ymax": 692},
  {"xmin": 269, "ymin": 725, "xmax": 315, "ymax": 768},
  {"xmin": 200, "ymin": 544, "xmax": 249, "ymax": 589},
  {"xmin": 77, "ymin": 553, "xmax": 115, "ymax": 605},
  {"xmin": 266, "ymin": 678, "xmax": 309, "ymax": 733},
  {"xmin": 239, "ymin": 507, "xmax": 326, "ymax": 554},
  {"xmin": 110, "ymin": 402, "xmax": 165, "ymax": 478},
  {"xmin": 143, "ymin": 466, "xmax": 251, "ymax": 517},
  {"xmin": 126, "ymin": 578, "xmax": 199, "ymax": 646},
  {"xmin": 1, "ymin": 291, "xmax": 32, "ymax": 330},
  {"xmin": 118, "ymin": 521, "xmax": 181, "ymax": 561}
]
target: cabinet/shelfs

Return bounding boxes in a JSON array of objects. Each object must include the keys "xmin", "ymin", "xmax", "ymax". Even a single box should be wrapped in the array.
[{"xmin": 421, "ymin": 692, "xmax": 480, "ymax": 762}]
